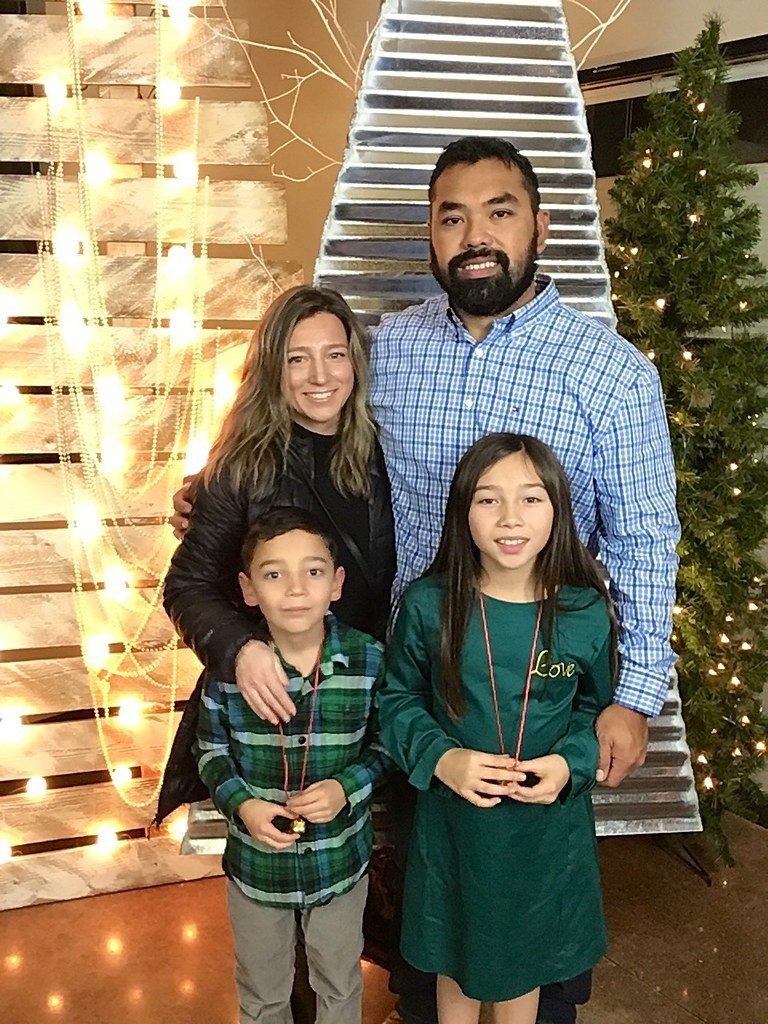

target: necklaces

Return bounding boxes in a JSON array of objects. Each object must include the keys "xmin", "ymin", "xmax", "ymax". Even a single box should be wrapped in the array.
[
  {"xmin": 480, "ymin": 577, "xmax": 545, "ymax": 785},
  {"xmin": 268, "ymin": 628, "xmax": 326, "ymax": 833},
  {"xmin": 155, "ymin": 136, "xmax": 682, "ymax": 1024}
]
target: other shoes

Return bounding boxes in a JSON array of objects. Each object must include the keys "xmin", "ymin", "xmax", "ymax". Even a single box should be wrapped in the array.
[{"xmin": 382, "ymin": 1011, "xmax": 405, "ymax": 1024}]
